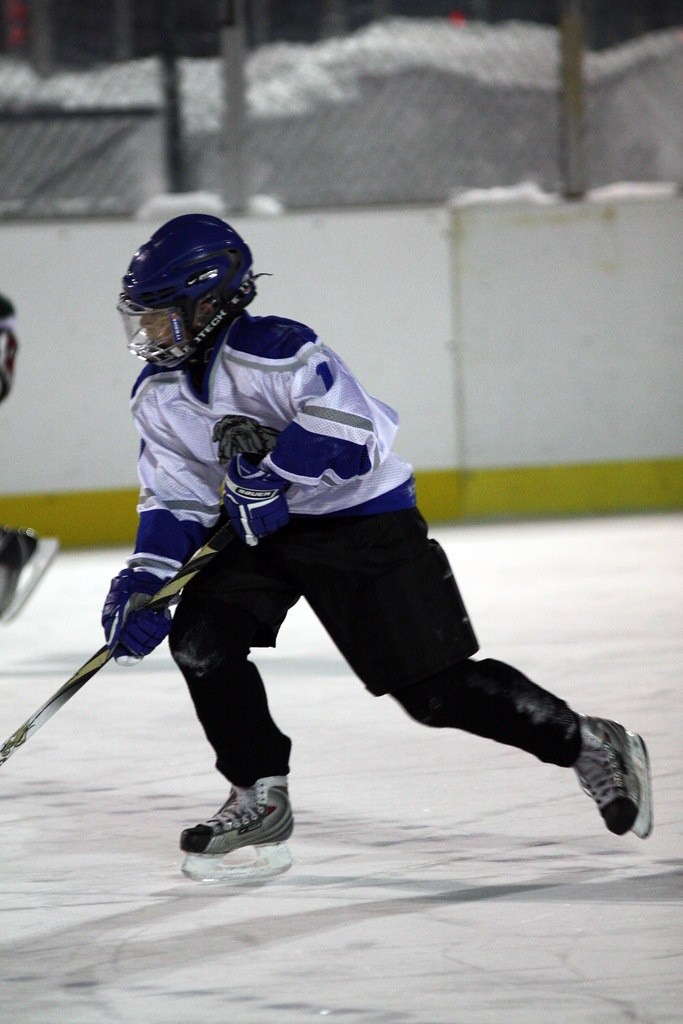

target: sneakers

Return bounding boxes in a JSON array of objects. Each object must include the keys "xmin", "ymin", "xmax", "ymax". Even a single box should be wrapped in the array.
[
  {"xmin": 571, "ymin": 712, "xmax": 655, "ymax": 840},
  {"xmin": 179, "ymin": 776, "xmax": 291, "ymax": 886},
  {"xmin": 0, "ymin": 528, "xmax": 60, "ymax": 620}
]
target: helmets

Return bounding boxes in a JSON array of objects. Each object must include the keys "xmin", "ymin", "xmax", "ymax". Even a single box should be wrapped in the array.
[{"xmin": 116, "ymin": 214, "xmax": 258, "ymax": 367}]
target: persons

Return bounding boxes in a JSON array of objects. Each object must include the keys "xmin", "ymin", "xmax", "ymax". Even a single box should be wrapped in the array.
[
  {"xmin": 0, "ymin": 296, "xmax": 58, "ymax": 620},
  {"xmin": 101, "ymin": 214, "xmax": 653, "ymax": 881}
]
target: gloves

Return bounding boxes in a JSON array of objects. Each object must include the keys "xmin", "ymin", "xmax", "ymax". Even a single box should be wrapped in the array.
[
  {"xmin": 219, "ymin": 452, "xmax": 289, "ymax": 546},
  {"xmin": 102, "ymin": 552, "xmax": 184, "ymax": 664}
]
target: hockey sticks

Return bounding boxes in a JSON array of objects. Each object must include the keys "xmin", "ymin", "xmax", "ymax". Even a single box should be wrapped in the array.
[{"xmin": 1, "ymin": 507, "xmax": 240, "ymax": 769}]
